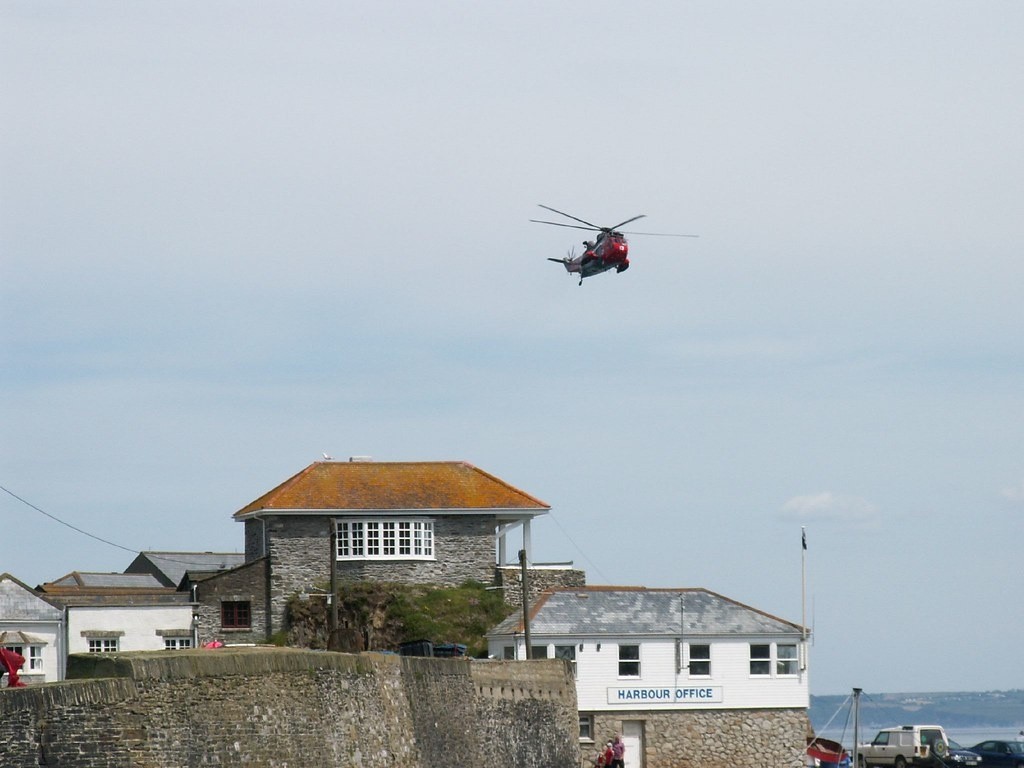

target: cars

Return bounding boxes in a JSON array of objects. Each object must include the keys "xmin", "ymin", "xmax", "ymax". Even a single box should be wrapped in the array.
[
  {"xmin": 968, "ymin": 739, "xmax": 1024, "ymax": 768},
  {"xmin": 947, "ymin": 738, "xmax": 983, "ymax": 768}
]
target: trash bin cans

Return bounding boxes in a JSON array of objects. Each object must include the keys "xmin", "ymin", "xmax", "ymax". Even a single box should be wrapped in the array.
[
  {"xmin": 433, "ymin": 644, "xmax": 467, "ymax": 657},
  {"xmin": 820, "ymin": 755, "xmax": 852, "ymax": 768},
  {"xmin": 398, "ymin": 639, "xmax": 434, "ymax": 656}
]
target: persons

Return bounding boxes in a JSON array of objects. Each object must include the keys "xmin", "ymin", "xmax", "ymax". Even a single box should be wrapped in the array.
[{"xmin": 598, "ymin": 735, "xmax": 625, "ymax": 768}]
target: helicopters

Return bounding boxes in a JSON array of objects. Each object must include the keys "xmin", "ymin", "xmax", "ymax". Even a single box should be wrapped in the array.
[{"xmin": 530, "ymin": 204, "xmax": 698, "ymax": 286}]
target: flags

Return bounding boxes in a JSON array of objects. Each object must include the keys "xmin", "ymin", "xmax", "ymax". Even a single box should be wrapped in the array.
[{"xmin": 801, "ymin": 533, "xmax": 808, "ymax": 549}]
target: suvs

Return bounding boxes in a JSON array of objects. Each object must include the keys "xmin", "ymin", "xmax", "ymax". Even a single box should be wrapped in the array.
[{"xmin": 856, "ymin": 726, "xmax": 948, "ymax": 768}]
